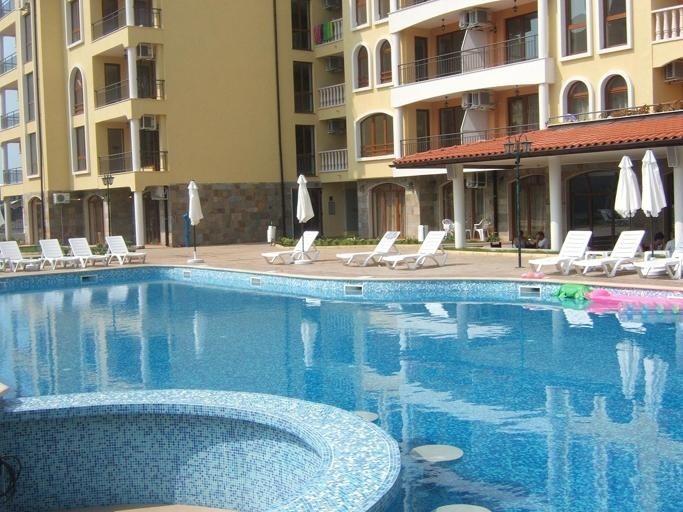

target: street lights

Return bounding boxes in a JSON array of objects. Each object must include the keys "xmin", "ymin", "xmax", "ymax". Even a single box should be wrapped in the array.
[
  {"xmin": 504, "ymin": 134, "xmax": 533, "ymax": 267},
  {"xmin": 102, "ymin": 171, "xmax": 115, "ymax": 236}
]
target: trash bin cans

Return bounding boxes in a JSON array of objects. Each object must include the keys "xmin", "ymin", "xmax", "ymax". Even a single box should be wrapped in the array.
[{"xmin": 267, "ymin": 225, "xmax": 276, "ymax": 246}]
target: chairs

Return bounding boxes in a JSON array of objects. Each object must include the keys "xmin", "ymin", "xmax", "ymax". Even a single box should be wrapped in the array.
[
  {"xmin": 599, "ymin": 209, "xmax": 628, "ymax": 226},
  {"xmin": 0, "ymin": 235, "xmax": 147, "ymax": 273},
  {"xmin": 441, "ymin": 218, "xmax": 456, "ymax": 240},
  {"xmin": 260, "ymin": 231, "xmax": 322, "ymax": 265},
  {"xmin": 473, "ymin": 216, "xmax": 491, "ymax": 242},
  {"xmin": 381, "ymin": 230, "xmax": 448, "ymax": 271},
  {"xmin": 335, "ymin": 230, "xmax": 400, "ymax": 267},
  {"xmin": 528, "ymin": 230, "xmax": 682, "ymax": 280}
]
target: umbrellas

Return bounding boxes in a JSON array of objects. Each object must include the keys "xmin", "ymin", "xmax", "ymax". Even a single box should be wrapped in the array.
[
  {"xmin": 187, "ymin": 180, "xmax": 205, "ymax": 258},
  {"xmin": 641, "ymin": 149, "xmax": 668, "ymax": 257},
  {"xmin": 614, "ymin": 155, "xmax": 642, "ymax": 230},
  {"xmin": 296, "ymin": 174, "xmax": 315, "ymax": 253},
  {"xmin": 299, "ymin": 304, "xmax": 320, "ymax": 370},
  {"xmin": 616, "ymin": 337, "xmax": 642, "ymax": 401},
  {"xmin": 643, "ymin": 350, "xmax": 669, "ymax": 422},
  {"xmin": 191, "ymin": 293, "xmax": 209, "ymax": 359}
]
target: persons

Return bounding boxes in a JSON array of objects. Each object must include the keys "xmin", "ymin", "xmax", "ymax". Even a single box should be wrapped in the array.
[
  {"xmin": 515, "ymin": 231, "xmax": 530, "ymax": 248},
  {"xmin": 650, "ymin": 231, "xmax": 665, "ymax": 250},
  {"xmin": 533, "ymin": 232, "xmax": 549, "ymax": 249}
]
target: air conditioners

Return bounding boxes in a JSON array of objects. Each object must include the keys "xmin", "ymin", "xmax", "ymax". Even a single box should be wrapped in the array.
[
  {"xmin": 150, "ymin": 186, "xmax": 164, "ymax": 198},
  {"xmin": 140, "ymin": 115, "xmax": 156, "ymax": 131},
  {"xmin": 328, "ymin": 119, "xmax": 345, "ymax": 134},
  {"xmin": 136, "ymin": 43, "xmax": 154, "ymax": 60},
  {"xmin": 325, "ymin": 57, "xmax": 343, "ymax": 72},
  {"xmin": 665, "ymin": 60, "xmax": 683, "ymax": 82},
  {"xmin": 458, "ymin": 8, "xmax": 494, "ymax": 31},
  {"xmin": 53, "ymin": 192, "xmax": 70, "ymax": 206},
  {"xmin": 466, "ymin": 171, "xmax": 487, "ymax": 189},
  {"xmin": 461, "ymin": 91, "xmax": 495, "ymax": 109},
  {"xmin": 323, "ymin": 0, "xmax": 341, "ymax": 10}
]
